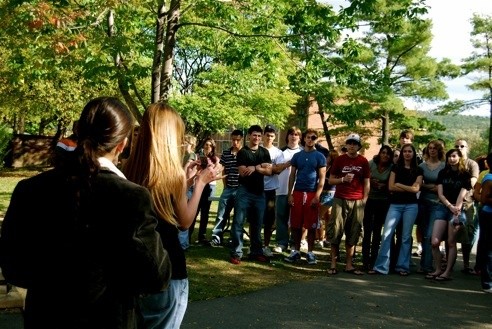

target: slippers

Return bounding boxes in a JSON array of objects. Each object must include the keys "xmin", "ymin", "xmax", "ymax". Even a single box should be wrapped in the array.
[
  {"xmin": 343, "ymin": 268, "xmax": 363, "ymax": 275},
  {"xmin": 435, "ymin": 275, "xmax": 455, "ymax": 281},
  {"xmin": 425, "ymin": 272, "xmax": 443, "ymax": 279},
  {"xmin": 400, "ymin": 271, "xmax": 409, "ymax": 276},
  {"xmin": 367, "ymin": 270, "xmax": 386, "ymax": 275},
  {"xmin": 327, "ymin": 267, "xmax": 339, "ymax": 275}
]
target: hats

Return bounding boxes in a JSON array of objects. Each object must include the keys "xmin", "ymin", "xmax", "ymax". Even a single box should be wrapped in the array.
[
  {"xmin": 345, "ymin": 132, "xmax": 361, "ymax": 145},
  {"xmin": 265, "ymin": 125, "xmax": 275, "ymax": 131}
]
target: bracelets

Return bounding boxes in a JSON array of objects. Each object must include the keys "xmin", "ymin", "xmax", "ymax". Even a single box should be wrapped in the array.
[{"xmin": 342, "ymin": 177, "xmax": 344, "ymax": 183}]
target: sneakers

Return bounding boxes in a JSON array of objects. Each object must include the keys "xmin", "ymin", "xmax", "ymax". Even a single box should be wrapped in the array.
[
  {"xmin": 285, "ymin": 250, "xmax": 302, "ymax": 264},
  {"xmin": 306, "ymin": 253, "xmax": 318, "ymax": 265}
]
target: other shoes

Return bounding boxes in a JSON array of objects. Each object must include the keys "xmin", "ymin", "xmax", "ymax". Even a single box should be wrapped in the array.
[
  {"xmin": 209, "ymin": 238, "xmax": 219, "ymax": 245},
  {"xmin": 272, "ymin": 245, "xmax": 287, "ymax": 252},
  {"xmin": 230, "ymin": 256, "xmax": 240, "ymax": 264},
  {"xmin": 199, "ymin": 238, "xmax": 211, "ymax": 246},
  {"xmin": 464, "ymin": 268, "xmax": 473, "ymax": 275},
  {"xmin": 481, "ymin": 282, "xmax": 492, "ymax": 293},
  {"xmin": 415, "ymin": 268, "xmax": 430, "ymax": 274},
  {"xmin": 263, "ymin": 248, "xmax": 273, "ymax": 256},
  {"xmin": 329, "ymin": 249, "xmax": 341, "ymax": 261},
  {"xmin": 249, "ymin": 252, "xmax": 268, "ymax": 262}
]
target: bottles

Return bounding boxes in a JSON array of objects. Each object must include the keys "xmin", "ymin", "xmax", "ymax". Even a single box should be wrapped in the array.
[{"xmin": 460, "ymin": 211, "xmax": 466, "ymax": 221}]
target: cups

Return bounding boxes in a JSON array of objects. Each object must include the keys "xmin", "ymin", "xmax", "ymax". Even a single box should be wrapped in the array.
[{"xmin": 348, "ymin": 173, "xmax": 355, "ymax": 178}]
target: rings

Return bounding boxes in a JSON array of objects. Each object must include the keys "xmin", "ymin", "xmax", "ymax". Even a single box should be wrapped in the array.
[{"xmin": 217, "ymin": 171, "xmax": 219, "ymax": 174}]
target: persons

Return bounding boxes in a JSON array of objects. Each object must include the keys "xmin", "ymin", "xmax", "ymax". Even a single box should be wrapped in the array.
[
  {"xmin": 124, "ymin": 123, "xmax": 492, "ymax": 283},
  {"xmin": 0, "ymin": 96, "xmax": 170, "ymax": 329},
  {"xmin": 53, "ymin": 121, "xmax": 80, "ymax": 165},
  {"xmin": 124, "ymin": 102, "xmax": 228, "ymax": 329}
]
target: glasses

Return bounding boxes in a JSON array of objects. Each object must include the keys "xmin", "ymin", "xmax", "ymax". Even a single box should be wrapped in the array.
[{"xmin": 454, "ymin": 145, "xmax": 464, "ymax": 149}]
